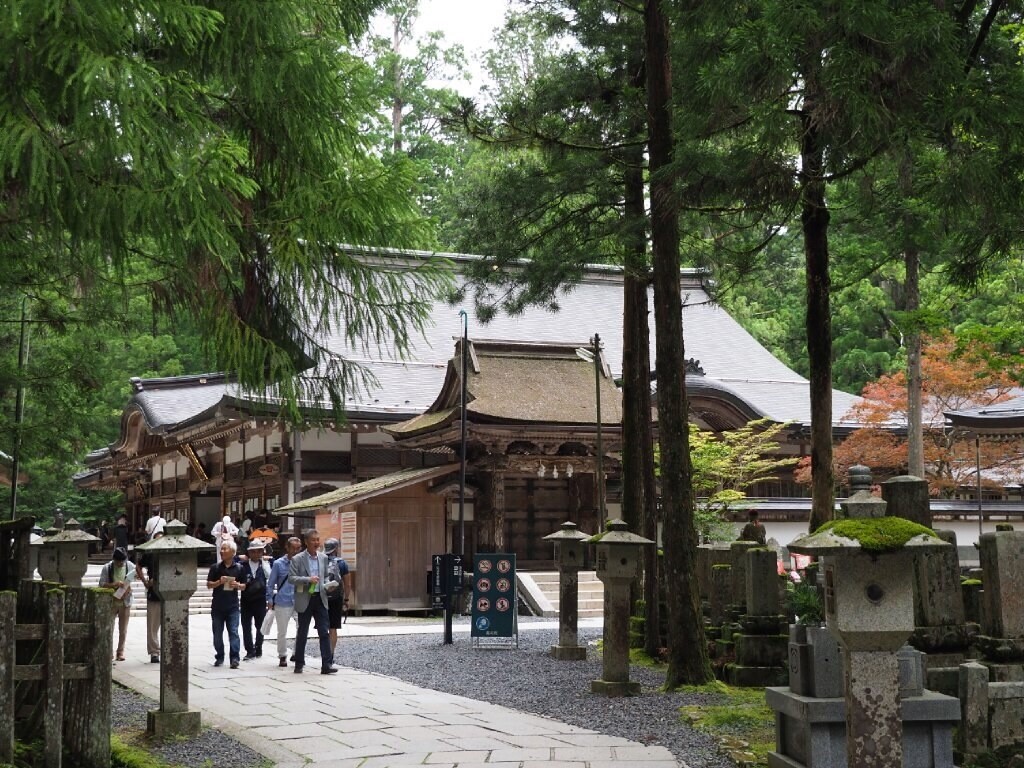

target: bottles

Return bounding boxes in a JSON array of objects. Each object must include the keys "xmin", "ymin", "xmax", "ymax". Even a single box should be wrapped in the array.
[{"xmin": 308, "ymin": 583, "xmax": 317, "ymax": 594}]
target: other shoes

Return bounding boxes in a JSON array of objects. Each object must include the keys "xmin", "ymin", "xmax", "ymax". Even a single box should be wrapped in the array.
[
  {"xmin": 294, "ymin": 663, "xmax": 303, "ymax": 674},
  {"xmin": 290, "ymin": 655, "xmax": 297, "ymax": 661},
  {"xmin": 117, "ymin": 653, "xmax": 124, "ymax": 660},
  {"xmin": 243, "ymin": 652, "xmax": 254, "ymax": 660},
  {"xmin": 255, "ymin": 644, "xmax": 263, "ymax": 657},
  {"xmin": 230, "ymin": 659, "xmax": 239, "ymax": 668},
  {"xmin": 321, "ymin": 666, "xmax": 338, "ymax": 674},
  {"xmin": 215, "ymin": 660, "xmax": 224, "ymax": 666},
  {"xmin": 151, "ymin": 656, "xmax": 159, "ymax": 663},
  {"xmin": 279, "ymin": 655, "xmax": 287, "ymax": 667}
]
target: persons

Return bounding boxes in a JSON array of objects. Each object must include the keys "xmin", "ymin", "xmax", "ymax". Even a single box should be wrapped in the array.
[
  {"xmin": 100, "ymin": 506, "xmax": 207, "ymax": 560},
  {"xmin": 135, "ymin": 532, "xmax": 164, "ymax": 663},
  {"xmin": 741, "ymin": 511, "xmax": 771, "ymax": 546},
  {"xmin": 98, "ymin": 547, "xmax": 138, "ymax": 660},
  {"xmin": 210, "ymin": 514, "xmax": 351, "ymax": 673}
]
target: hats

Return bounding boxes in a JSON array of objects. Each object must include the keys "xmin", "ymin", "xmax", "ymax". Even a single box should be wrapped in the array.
[
  {"xmin": 247, "ymin": 541, "xmax": 264, "ymax": 550},
  {"xmin": 223, "ymin": 516, "xmax": 231, "ymax": 527},
  {"xmin": 112, "ymin": 547, "xmax": 127, "ymax": 561},
  {"xmin": 324, "ymin": 538, "xmax": 339, "ymax": 555}
]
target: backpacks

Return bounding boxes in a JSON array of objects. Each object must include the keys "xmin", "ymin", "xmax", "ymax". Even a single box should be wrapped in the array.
[{"xmin": 324, "ymin": 556, "xmax": 344, "ymax": 591}]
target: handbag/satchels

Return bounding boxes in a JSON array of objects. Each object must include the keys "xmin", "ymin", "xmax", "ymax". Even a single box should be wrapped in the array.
[
  {"xmin": 271, "ymin": 594, "xmax": 277, "ymax": 610},
  {"xmin": 260, "ymin": 609, "xmax": 276, "ymax": 635}
]
